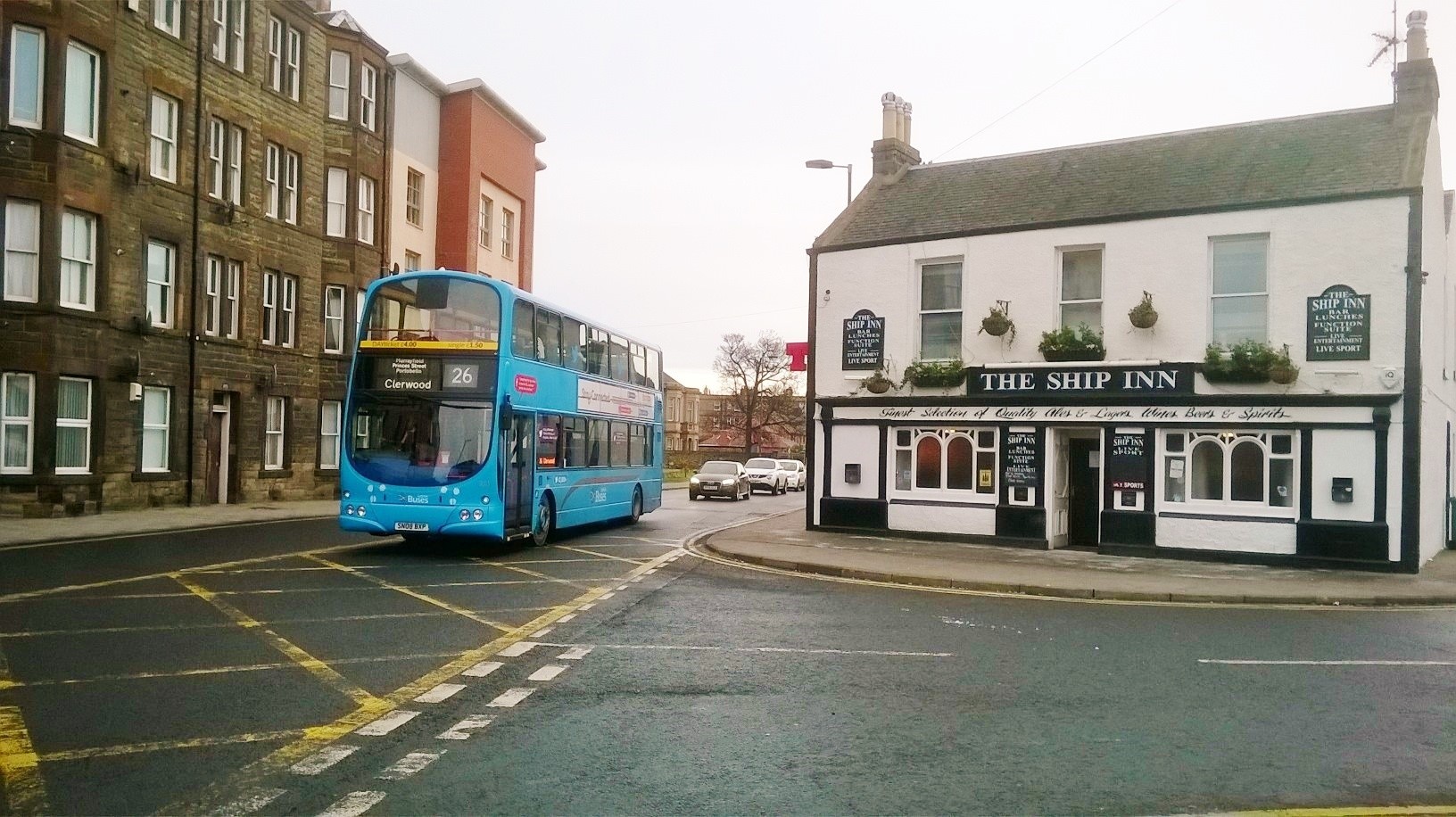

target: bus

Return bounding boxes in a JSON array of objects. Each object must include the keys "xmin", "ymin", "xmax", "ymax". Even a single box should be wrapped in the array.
[{"xmin": 338, "ymin": 270, "xmax": 665, "ymax": 549}]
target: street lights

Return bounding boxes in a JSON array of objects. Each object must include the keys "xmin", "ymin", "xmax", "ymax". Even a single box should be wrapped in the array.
[
  {"xmin": 805, "ymin": 159, "xmax": 852, "ymax": 208},
  {"xmin": 759, "ymin": 355, "xmax": 778, "ymax": 458}
]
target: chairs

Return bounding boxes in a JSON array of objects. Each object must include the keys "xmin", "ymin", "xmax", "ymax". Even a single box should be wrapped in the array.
[{"xmin": 391, "ymin": 337, "xmax": 402, "ymax": 341}]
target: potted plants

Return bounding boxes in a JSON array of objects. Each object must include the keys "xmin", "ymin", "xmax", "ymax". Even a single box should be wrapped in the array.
[
  {"xmin": 1038, "ymin": 322, "xmax": 1106, "ymax": 361},
  {"xmin": 977, "ymin": 306, "xmax": 1016, "ymax": 352},
  {"xmin": 1198, "ymin": 338, "xmax": 1301, "ymax": 385},
  {"xmin": 1126, "ymin": 290, "xmax": 1159, "ymax": 335},
  {"xmin": 856, "ymin": 352, "xmax": 967, "ymax": 396}
]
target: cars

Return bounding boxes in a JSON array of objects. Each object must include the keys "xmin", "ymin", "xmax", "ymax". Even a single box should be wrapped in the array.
[
  {"xmin": 743, "ymin": 457, "xmax": 789, "ymax": 496},
  {"xmin": 776, "ymin": 459, "xmax": 806, "ymax": 492},
  {"xmin": 689, "ymin": 460, "xmax": 751, "ymax": 502}
]
